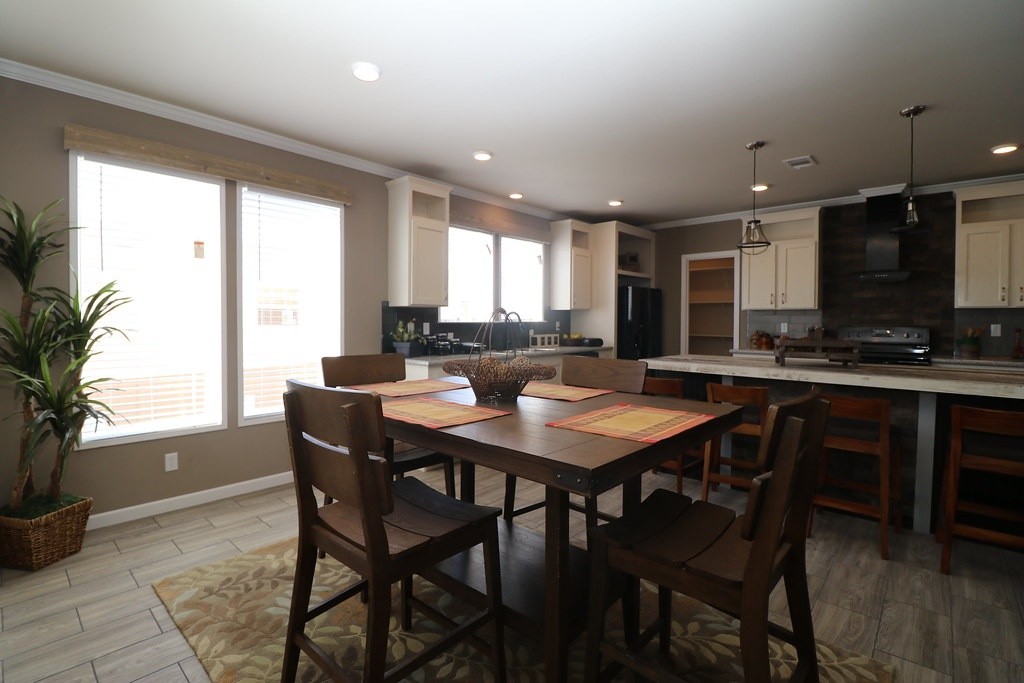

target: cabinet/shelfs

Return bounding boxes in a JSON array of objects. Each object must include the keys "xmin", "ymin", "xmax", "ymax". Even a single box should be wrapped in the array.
[
  {"xmin": 953, "ymin": 180, "xmax": 1024, "ymax": 309},
  {"xmin": 550, "ymin": 220, "xmax": 656, "ymax": 359},
  {"xmin": 688, "ymin": 258, "xmax": 733, "ymax": 355},
  {"xmin": 385, "ymin": 175, "xmax": 454, "ymax": 308},
  {"xmin": 741, "ymin": 207, "xmax": 822, "ymax": 310}
]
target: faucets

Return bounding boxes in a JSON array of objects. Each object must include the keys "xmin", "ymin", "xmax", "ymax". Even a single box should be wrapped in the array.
[{"xmin": 506, "ymin": 311, "xmax": 523, "ymax": 350}]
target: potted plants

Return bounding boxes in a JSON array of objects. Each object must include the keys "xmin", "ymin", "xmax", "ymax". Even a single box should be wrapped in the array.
[{"xmin": 0, "ymin": 192, "xmax": 134, "ymax": 573}]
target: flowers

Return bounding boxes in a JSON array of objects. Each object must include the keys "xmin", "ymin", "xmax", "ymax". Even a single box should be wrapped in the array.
[{"xmin": 389, "ymin": 318, "xmax": 427, "ymax": 346}]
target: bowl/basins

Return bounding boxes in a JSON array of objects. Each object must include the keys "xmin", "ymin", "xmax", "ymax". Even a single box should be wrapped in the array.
[
  {"xmin": 562, "ymin": 338, "xmax": 583, "ymax": 346},
  {"xmin": 582, "ymin": 338, "xmax": 603, "ymax": 347}
]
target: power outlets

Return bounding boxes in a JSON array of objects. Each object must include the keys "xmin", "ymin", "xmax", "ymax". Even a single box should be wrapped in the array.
[
  {"xmin": 165, "ymin": 452, "xmax": 178, "ymax": 472},
  {"xmin": 991, "ymin": 324, "xmax": 1002, "ymax": 337},
  {"xmin": 556, "ymin": 321, "xmax": 561, "ymax": 331},
  {"xmin": 780, "ymin": 323, "xmax": 788, "ymax": 333}
]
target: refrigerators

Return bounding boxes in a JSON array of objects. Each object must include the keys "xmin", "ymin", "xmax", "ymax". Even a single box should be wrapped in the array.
[{"xmin": 617, "ymin": 286, "xmax": 663, "ymax": 360}]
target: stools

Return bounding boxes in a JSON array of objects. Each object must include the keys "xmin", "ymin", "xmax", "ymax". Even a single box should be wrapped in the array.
[
  {"xmin": 937, "ymin": 405, "xmax": 1024, "ymax": 575},
  {"xmin": 702, "ymin": 383, "xmax": 769, "ymax": 505},
  {"xmin": 806, "ymin": 384, "xmax": 904, "ymax": 560},
  {"xmin": 644, "ymin": 378, "xmax": 704, "ymax": 495}
]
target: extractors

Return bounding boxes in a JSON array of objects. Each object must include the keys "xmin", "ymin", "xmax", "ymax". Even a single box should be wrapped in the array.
[{"xmin": 839, "ymin": 193, "xmax": 926, "ymax": 284}]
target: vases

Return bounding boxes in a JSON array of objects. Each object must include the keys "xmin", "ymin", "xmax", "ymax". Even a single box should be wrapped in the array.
[{"xmin": 393, "ymin": 341, "xmax": 425, "ymax": 357}]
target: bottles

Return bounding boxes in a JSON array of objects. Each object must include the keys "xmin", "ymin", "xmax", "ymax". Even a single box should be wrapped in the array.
[
  {"xmin": 750, "ymin": 329, "xmax": 760, "ymax": 349},
  {"xmin": 1011, "ymin": 327, "xmax": 1024, "ymax": 360},
  {"xmin": 760, "ymin": 334, "xmax": 772, "ymax": 349}
]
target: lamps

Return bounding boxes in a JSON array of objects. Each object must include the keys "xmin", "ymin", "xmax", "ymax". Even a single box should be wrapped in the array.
[
  {"xmin": 898, "ymin": 103, "xmax": 925, "ymax": 227},
  {"xmin": 737, "ymin": 141, "xmax": 770, "ymax": 256}
]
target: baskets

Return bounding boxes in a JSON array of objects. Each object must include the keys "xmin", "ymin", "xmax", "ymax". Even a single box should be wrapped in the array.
[
  {"xmin": 0, "ymin": 491, "xmax": 95, "ymax": 572},
  {"xmin": 442, "ymin": 306, "xmax": 556, "ymax": 404}
]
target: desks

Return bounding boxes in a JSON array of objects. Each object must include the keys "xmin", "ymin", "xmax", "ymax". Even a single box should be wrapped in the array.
[{"xmin": 351, "ymin": 374, "xmax": 745, "ymax": 683}]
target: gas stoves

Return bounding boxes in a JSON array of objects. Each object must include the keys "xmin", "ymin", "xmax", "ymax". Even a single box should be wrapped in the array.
[{"xmin": 838, "ymin": 324, "xmax": 931, "ymax": 361}]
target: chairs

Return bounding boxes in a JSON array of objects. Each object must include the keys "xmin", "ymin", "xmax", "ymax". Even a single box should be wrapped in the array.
[
  {"xmin": 317, "ymin": 352, "xmax": 648, "ymax": 559},
  {"xmin": 278, "ymin": 381, "xmax": 509, "ymax": 683},
  {"xmin": 583, "ymin": 383, "xmax": 831, "ymax": 683}
]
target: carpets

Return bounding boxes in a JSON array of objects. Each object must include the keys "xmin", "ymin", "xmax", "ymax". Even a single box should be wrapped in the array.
[{"xmin": 153, "ymin": 536, "xmax": 898, "ymax": 683}]
